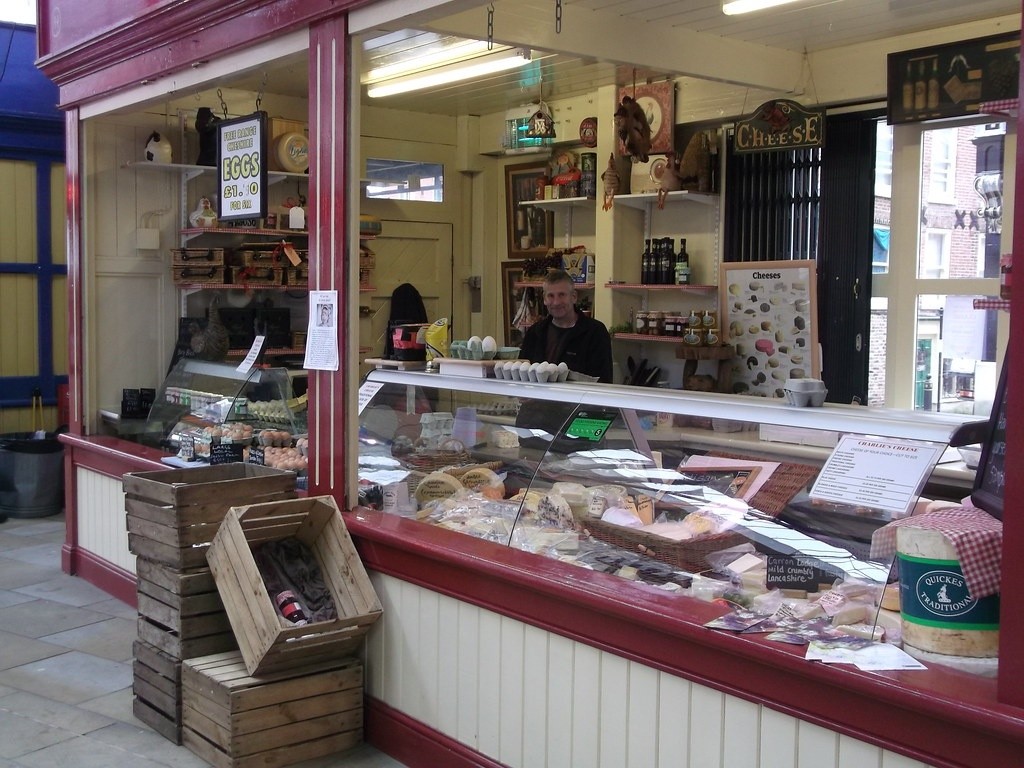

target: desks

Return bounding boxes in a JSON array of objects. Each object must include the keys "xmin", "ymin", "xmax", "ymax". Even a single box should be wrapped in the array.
[{"xmin": 720, "ymin": 0, "xmax": 797, "ymax": 16}]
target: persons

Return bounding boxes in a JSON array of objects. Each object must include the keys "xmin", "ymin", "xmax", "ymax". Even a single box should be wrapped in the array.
[
  {"xmin": 519, "ymin": 271, "xmax": 614, "ymax": 451},
  {"xmin": 318, "ymin": 307, "xmax": 330, "ymax": 327}
]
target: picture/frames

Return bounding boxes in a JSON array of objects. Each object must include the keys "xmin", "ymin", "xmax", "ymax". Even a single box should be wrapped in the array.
[
  {"xmin": 505, "ymin": 161, "xmax": 554, "ymax": 258},
  {"xmin": 501, "ymin": 261, "xmax": 546, "ymax": 348}
]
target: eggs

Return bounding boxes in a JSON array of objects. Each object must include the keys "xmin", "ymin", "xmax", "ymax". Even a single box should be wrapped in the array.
[
  {"xmin": 179, "ymin": 397, "xmax": 308, "ymax": 471},
  {"xmin": 494, "ymin": 360, "xmax": 568, "ymax": 374},
  {"xmin": 467, "ymin": 336, "xmax": 497, "ymax": 352}
]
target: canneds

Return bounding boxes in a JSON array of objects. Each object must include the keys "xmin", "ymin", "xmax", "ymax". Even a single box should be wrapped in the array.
[
  {"xmin": 534, "ymin": 175, "xmax": 549, "ymax": 200},
  {"xmin": 635, "ymin": 309, "xmax": 723, "ymax": 346},
  {"xmin": 277, "ymin": 590, "xmax": 306, "ymax": 623}
]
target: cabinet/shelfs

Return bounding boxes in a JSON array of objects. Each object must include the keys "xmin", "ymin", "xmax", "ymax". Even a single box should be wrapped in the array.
[
  {"xmin": 515, "ymin": 196, "xmax": 593, "ymax": 325},
  {"xmin": 605, "ymin": 190, "xmax": 719, "ymax": 342},
  {"xmin": 125, "ymin": 108, "xmax": 373, "ymax": 355}
]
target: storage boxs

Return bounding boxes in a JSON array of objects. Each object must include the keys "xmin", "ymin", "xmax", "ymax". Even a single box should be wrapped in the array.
[
  {"xmin": 205, "ymin": 493, "xmax": 385, "ymax": 677},
  {"xmin": 391, "ymin": 322, "xmax": 430, "ymax": 361},
  {"xmin": 123, "ymin": 462, "xmax": 297, "ymax": 569},
  {"xmin": 131, "ymin": 638, "xmax": 183, "ymax": 745},
  {"xmin": 760, "ymin": 423, "xmax": 841, "ymax": 448},
  {"xmin": 136, "ymin": 555, "xmax": 239, "ymax": 662},
  {"xmin": 179, "ymin": 650, "xmax": 365, "ymax": 768}
]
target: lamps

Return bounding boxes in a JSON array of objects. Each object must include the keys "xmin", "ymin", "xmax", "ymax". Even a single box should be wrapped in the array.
[{"xmin": 368, "ymin": 47, "xmax": 535, "ymax": 99}]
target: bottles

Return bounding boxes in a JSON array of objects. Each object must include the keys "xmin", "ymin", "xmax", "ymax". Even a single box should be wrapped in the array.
[
  {"xmin": 677, "ymin": 238, "xmax": 690, "ymax": 284},
  {"xmin": 276, "ymin": 591, "xmax": 305, "ymax": 622},
  {"xmin": 640, "ymin": 237, "xmax": 675, "ymax": 284}
]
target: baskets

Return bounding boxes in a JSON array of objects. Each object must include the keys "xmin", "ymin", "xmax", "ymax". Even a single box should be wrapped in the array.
[
  {"xmin": 588, "ymin": 505, "xmax": 747, "ymax": 574},
  {"xmin": 404, "ymin": 438, "xmax": 469, "ymax": 498}
]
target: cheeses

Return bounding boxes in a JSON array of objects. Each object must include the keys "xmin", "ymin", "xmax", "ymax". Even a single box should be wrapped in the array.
[{"xmin": 413, "ymin": 466, "xmax": 904, "ymax": 640}]
